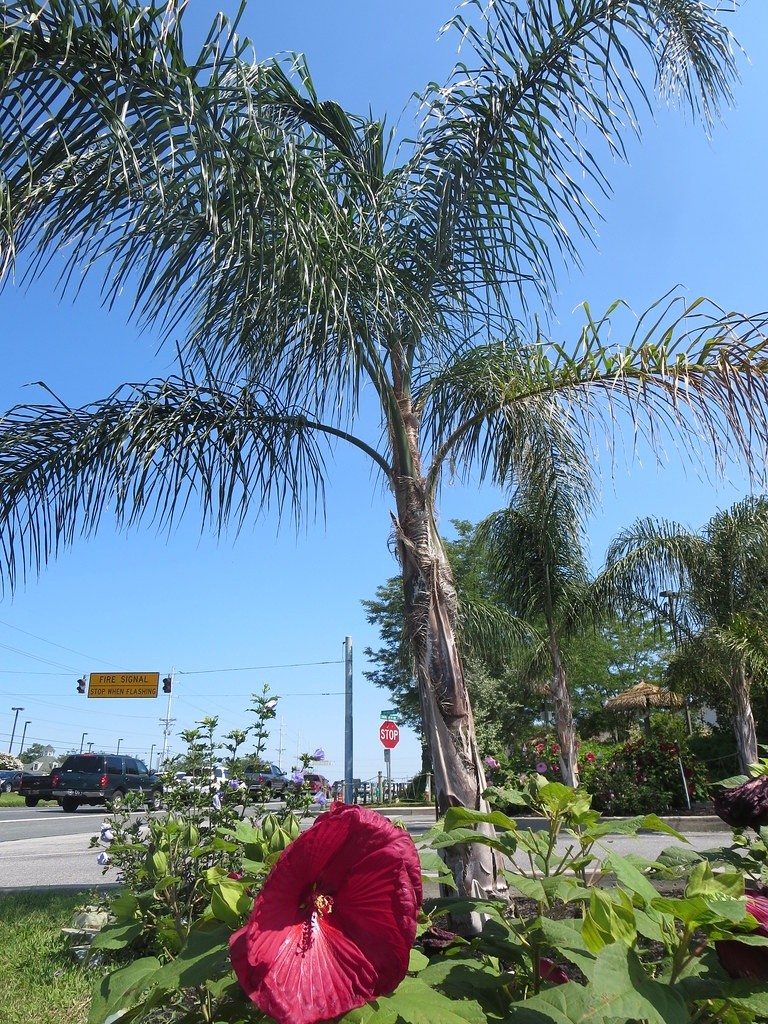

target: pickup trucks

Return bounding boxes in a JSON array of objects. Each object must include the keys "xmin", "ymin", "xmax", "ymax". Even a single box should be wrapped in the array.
[
  {"xmin": 16, "ymin": 768, "xmax": 60, "ymax": 808},
  {"xmin": 244, "ymin": 764, "xmax": 291, "ymax": 803},
  {"xmin": 301, "ymin": 774, "xmax": 332, "ymax": 798}
]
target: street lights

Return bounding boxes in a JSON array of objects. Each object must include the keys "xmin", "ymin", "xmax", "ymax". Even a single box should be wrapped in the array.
[
  {"xmin": 88, "ymin": 741, "xmax": 94, "ymax": 751},
  {"xmin": 19, "ymin": 721, "xmax": 32, "ymax": 752},
  {"xmin": 8, "ymin": 706, "xmax": 24, "ymax": 752},
  {"xmin": 116, "ymin": 738, "xmax": 123, "ymax": 755},
  {"xmin": 660, "ymin": 590, "xmax": 693, "ymax": 737},
  {"xmin": 80, "ymin": 732, "xmax": 88, "ymax": 755},
  {"xmin": 150, "ymin": 743, "xmax": 156, "ymax": 771}
]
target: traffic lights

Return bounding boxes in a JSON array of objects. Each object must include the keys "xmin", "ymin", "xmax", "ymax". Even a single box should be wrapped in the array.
[
  {"xmin": 76, "ymin": 678, "xmax": 87, "ymax": 694},
  {"xmin": 162, "ymin": 677, "xmax": 172, "ymax": 693}
]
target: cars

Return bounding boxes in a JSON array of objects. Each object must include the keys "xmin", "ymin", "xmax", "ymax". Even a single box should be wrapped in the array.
[
  {"xmin": 331, "ymin": 779, "xmax": 376, "ymax": 797},
  {"xmin": 154, "ymin": 771, "xmax": 190, "ymax": 795},
  {"xmin": 0, "ymin": 770, "xmax": 34, "ymax": 794}
]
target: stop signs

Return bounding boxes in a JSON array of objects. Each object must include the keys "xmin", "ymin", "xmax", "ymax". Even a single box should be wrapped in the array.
[{"xmin": 378, "ymin": 721, "xmax": 400, "ymax": 748}]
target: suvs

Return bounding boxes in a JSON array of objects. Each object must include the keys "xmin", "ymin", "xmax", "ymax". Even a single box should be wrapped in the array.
[
  {"xmin": 52, "ymin": 752, "xmax": 164, "ymax": 814},
  {"xmin": 180, "ymin": 766, "xmax": 248, "ymax": 808}
]
